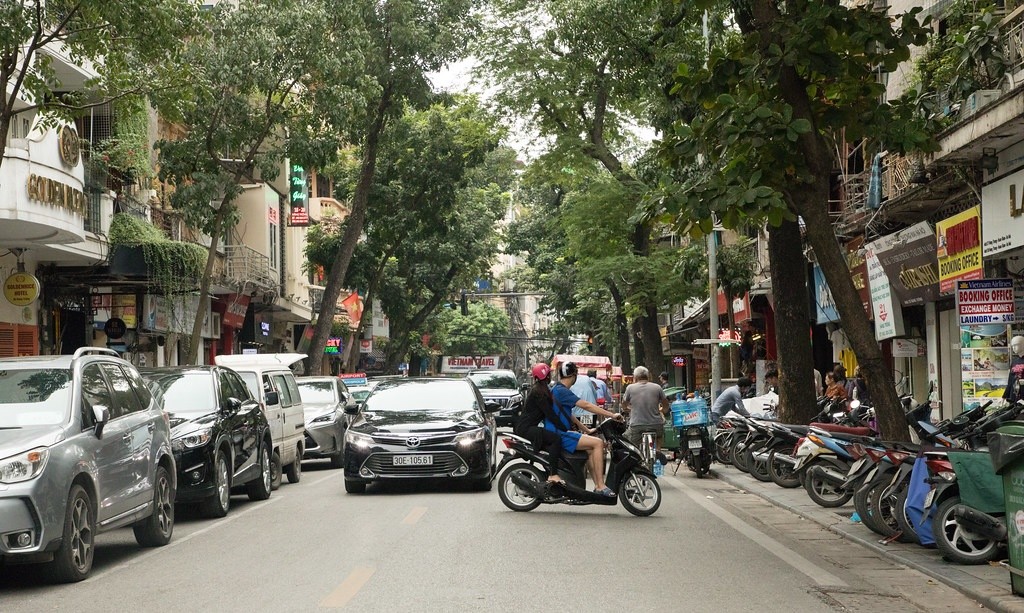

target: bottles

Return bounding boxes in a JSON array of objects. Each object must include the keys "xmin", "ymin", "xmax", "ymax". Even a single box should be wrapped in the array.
[
  {"xmin": 687, "ymin": 391, "xmax": 708, "ymax": 423},
  {"xmin": 671, "ymin": 393, "xmax": 688, "ymax": 425},
  {"xmin": 652, "ymin": 459, "xmax": 664, "ymax": 476}
]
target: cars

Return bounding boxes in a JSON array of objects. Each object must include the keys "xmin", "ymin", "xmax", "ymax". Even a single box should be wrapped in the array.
[
  {"xmin": 340, "ymin": 373, "xmax": 405, "ymax": 412},
  {"xmin": 342, "ymin": 376, "xmax": 501, "ymax": 495},
  {"xmin": 135, "ymin": 366, "xmax": 274, "ymax": 520},
  {"xmin": 262, "ymin": 375, "xmax": 360, "ymax": 469}
]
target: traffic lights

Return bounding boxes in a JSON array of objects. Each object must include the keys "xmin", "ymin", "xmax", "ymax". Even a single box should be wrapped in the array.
[{"xmin": 587, "ymin": 337, "xmax": 592, "ymax": 352}]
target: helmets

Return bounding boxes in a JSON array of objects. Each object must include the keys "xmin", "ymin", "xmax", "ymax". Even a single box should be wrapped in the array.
[
  {"xmin": 559, "ymin": 361, "xmax": 578, "ymax": 378},
  {"xmin": 532, "ymin": 362, "xmax": 554, "ymax": 381}
]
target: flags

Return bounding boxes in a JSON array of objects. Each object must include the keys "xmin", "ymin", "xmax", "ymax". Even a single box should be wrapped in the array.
[{"xmin": 342, "ymin": 292, "xmax": 361, "ymax": 323}]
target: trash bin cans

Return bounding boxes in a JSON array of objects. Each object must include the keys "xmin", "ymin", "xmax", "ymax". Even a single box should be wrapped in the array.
[
  {"xmin": 659, "ymin": 387, "xmax": 686, "ymax": 451},
  {"xmin": 995, "ymin": 420, "xmax": 1024, "ymax": 596}
]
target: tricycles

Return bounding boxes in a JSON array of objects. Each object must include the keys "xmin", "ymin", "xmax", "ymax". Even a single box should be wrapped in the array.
[{"xmin": 549, "ymin": 354, "xmax": 633, "ymax": 426}]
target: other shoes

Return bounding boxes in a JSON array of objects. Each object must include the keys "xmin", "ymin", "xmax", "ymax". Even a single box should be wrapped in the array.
[{"xmin": 656, "ymin": 451, "xmax": 667, "ymax": 465}]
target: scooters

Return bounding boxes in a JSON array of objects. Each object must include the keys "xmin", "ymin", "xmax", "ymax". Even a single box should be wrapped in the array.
[{"xmin": 672, "ymin": 423, "xmax": 713, "ymax": 479}]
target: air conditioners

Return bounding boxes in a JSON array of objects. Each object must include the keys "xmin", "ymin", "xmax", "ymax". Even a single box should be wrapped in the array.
[{"xmin": 960, "ymin": 90, "xmax": 1001, "ymax": 119}]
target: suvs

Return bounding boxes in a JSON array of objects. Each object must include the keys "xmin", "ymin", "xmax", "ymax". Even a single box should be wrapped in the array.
[
  {"xmin": 463, "ymin": 369, "xmax": 531, "ymax": 427},
  {"xmin": 0, "ymin": 347, "xmax": 179, "ymax": 583}
]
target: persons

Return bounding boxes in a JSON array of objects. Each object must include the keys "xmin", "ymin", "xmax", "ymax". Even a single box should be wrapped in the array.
[
  {"xmin": 515, "ymin": 363, "xmax": 564, "ymax": 487},
  {"xmin": 814, "ymin": 368, "xmax": 823, "ymax": 399},
  {"xmin": 587, "ymin": 370, "xmax": 613, "ymax": 403},
  {"xmin": 710, "ymin": 377, "xmax": 751, "ymax": 461},
  {"xmin": 545, "ymin": 362, "xmax": 625, "ymax": 498},
  {"xmin": 1005, "ymin": 336, "xmax": 1024, "ymax": 405},
  {"xmin": 834, "ymin": 365, "xmax": 870, "ymax": 401},
  {"xmin": 825, "ymin": 372, "xmax": 852, "ymax": 413},
  {"xmin": 658, "ymin": 372, "xmax": 674, "ymax": 399},
  {"xmin": 570, "ymin": 375, "xmax": 596, "ymax": 427},
  {"xmin": 622, "ymin": 366, "xmax": 669, "ymax": 464},
  {"xmin": 765, "ymin": 370, "xmax": 778, "ymax": 394}
]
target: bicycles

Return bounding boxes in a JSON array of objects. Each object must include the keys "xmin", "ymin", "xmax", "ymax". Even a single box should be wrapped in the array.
[{"xmin": 623, "ymin": 410, "xmax": 673, "ymax": 475}]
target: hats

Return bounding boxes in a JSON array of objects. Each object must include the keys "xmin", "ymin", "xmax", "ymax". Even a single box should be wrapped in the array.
[
  {"xmin": 587, "ymin": 369, "xmax": 596, "ymax": 377},
  {"xmin": 659, "ymin": 372, "xmax": 668, "ymax": 378}
]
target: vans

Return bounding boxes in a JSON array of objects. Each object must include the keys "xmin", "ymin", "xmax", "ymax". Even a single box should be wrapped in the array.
[{"xmin": 212, "ymin": 352, "xmax": 309, "ymax": 491}]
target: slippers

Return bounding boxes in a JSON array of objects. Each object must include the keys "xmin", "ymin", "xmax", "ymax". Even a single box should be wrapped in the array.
[
  {"xmin": 593, "ymin": 487, "xmax": 616, "ymax": 497},
  {"xmin": 546, "ymin": 479, "xmax": 566, "ymax": 486}
]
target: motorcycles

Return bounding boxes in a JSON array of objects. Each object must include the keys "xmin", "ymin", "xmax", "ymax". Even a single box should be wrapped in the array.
[
  {"xmin": 708, "ymin": 380, "xmax": 1024, "ymax": 564},
  {"xmin": 488, "ymin": 411, "xmax": 663, "ymax": 517}
]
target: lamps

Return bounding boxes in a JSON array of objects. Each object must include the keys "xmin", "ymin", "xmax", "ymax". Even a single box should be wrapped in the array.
[
  {"xmin": 982, "ymin": 147, "xmax": 998, "ymax": 169},
  {"xmin": 910, "ymin": 159, "xmax": 929, "ymax": 181}
]
